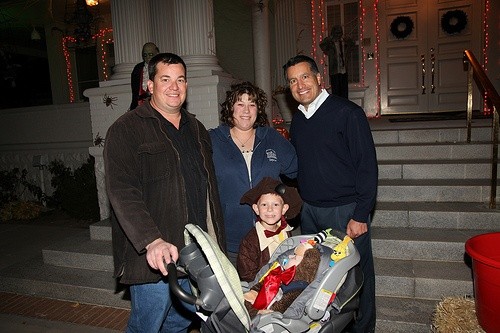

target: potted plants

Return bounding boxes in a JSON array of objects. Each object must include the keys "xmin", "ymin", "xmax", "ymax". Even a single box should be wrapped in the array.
[{"xmin": 319, "ymin": 24, "xmax": 358, "ymax": 98}]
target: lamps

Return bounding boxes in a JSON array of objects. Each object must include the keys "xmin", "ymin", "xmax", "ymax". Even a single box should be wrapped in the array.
[{"xmin": 30, "ymin": 23, "xmax": 41, "ymax": 41}]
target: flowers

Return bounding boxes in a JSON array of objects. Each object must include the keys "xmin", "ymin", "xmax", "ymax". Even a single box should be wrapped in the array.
[{"xmin": 271, "ymin": 84, "xmax": 291, "ymax": 97}]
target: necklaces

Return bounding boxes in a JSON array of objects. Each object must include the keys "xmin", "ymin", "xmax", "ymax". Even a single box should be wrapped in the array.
[{"xmin": 235, "ymin": 135, "xmax": 252, "ymax": 147}]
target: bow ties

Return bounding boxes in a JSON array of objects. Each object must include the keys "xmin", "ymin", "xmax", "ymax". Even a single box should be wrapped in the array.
[{"xmin": 254, "ymin": 264, "xmax": 297, "ymax": 310}]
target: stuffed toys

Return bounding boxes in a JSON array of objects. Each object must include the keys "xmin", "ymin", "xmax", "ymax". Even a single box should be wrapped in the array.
[{"xmin": 242, "ymin": 228, "xmax": 333, "ymax": 319}]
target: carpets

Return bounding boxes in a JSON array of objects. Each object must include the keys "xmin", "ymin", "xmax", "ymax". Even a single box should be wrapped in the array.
[{"xmin": 388, "ymin": 114, "xmax": 494, "ymax": 123}]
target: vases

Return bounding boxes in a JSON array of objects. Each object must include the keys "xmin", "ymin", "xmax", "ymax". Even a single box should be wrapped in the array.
[{"xmin": 273, "ymin": 93, "xmax": 299, "ymax": 127}]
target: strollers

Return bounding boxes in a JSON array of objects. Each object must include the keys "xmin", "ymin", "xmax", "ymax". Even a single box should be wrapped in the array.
[{"xmin": 167, "ymin": 223, "xmax": 366, "ymax": 333}]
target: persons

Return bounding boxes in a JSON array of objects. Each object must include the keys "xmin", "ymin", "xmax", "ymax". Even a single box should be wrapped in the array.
[
  {"xmin": 130, "ymin": 43, "xmax": 160, "ymax": 109},
  {"xmin": 103, "ymin": 54, "xmax": 225, "ymax": 333},
  {"xmin": 282, "ymin": 56, "xmax": 379, "ymax": 333},
  {"xmin": 207, "ymin": 82, "xmax": 297, "ymax": 270},
  {"xmin": 238, "ymin": 177, "xmax": 303, "ymax": 282}
]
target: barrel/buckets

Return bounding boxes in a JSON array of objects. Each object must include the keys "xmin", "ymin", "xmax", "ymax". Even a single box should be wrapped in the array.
[{"xmin": 465, "ymin": 231, "xmax": 500, "ymax": 333}]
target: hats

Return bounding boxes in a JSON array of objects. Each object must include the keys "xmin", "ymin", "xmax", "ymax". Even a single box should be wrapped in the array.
[{"xmin": 241, "ymin": 178, "xmax": 302, "ymax": 219}]
